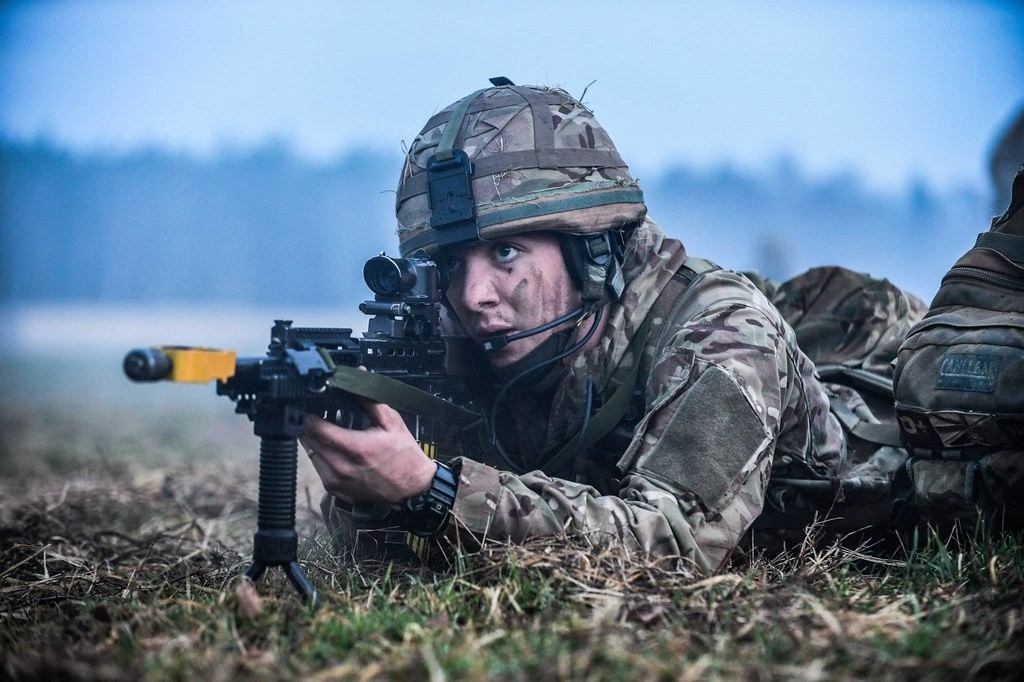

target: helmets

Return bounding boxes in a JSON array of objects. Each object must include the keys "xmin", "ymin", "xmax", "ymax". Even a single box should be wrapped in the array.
[{"xmin": 395, "ymin": 85, "xmax": 647, "ymax": 258}]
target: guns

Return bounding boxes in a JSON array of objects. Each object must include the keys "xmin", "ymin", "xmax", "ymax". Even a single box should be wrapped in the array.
[{"xmin": 124, "ymin": 250, "xmax": 507, "ymax": 608}]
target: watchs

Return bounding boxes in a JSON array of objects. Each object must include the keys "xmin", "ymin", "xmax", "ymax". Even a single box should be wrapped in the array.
[{"xmin": 397, "ymin": 459, "xmax": 457, "ymax": 540}]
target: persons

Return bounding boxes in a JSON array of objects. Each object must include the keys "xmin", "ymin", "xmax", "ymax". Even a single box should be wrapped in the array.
[
  {"xmin": 892, "ymin": 161, "xmax": 1024, "ymax": 533},
  {"xmin": 300, "ymin": 77, "xmax": 934, "ymax": 578}
]
